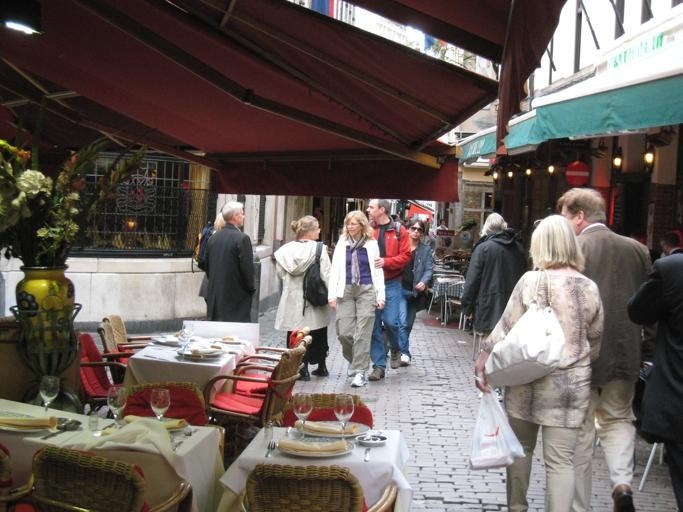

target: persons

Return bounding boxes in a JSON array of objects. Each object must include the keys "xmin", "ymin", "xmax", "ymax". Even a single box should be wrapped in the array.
[
  {"xmin": 660, "ymin": 233, "xmax": 683, "ymax": 256},
  {"xmin": 274, "ymin": 214, "xmax": 332, "ymax": 381},
  {"xmin": 557, "ymin": 188, "xmax": 659, "ymax": 511},
  {"xmin": 366, "ymin": 199, "xmax": 412, "ymax": 380},
  {"xmin": 199, "ymin": 213, "xmax": 227, "ymax": 304},
  {"xmin": 474, "ymin": 214, "xmax": 605, "ymax": 512},
  {"xmin": 434, "ymin": 219, "xmax": 448, "ymax": 236},
  {"xmin": 328, "ymin": 210, "xmax": 386, "ymax": 387},
  {"xmin": 627, "ymin": 254, "xmax": 683, "ymax": 511},
  {"xmin": 382, "ymin": 220, "xmax": 433, "ymax": 366},
  {"xmin": 461, "ymin": 212, "xmax": 527, "ymax": 403},
  {"xmin": 391, "ymin": 215, "xmax": 400, "ymax": 222},
  {"xmin": 203, "ymin": 200, "xmax": 257, "ymax": 323}
]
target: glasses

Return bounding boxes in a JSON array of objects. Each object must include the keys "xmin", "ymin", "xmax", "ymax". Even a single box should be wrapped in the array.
[{"xmin": 411, "ymin": 227, "xmax": 423, "ymax": 232}]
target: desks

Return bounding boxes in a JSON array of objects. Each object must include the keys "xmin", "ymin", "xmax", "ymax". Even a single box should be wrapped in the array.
[{"xmin": 432, "ymin": 276, "xmax": 463, "ymax": 319}]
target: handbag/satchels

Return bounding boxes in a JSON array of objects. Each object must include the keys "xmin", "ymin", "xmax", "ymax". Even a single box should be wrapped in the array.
[{"xmin": 485, "ymin": 301, "xmax": 565, "ymax": 387}]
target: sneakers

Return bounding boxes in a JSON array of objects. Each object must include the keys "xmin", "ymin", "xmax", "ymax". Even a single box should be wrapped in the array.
[{"xmin": 346, "ymin": 351, "xmax": 412, "ymax": 387}]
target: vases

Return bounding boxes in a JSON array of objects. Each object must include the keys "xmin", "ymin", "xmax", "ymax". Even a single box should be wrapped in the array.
[{"xmin": 16, "ymin": 266, "xmax": 75, "ymax": 367}]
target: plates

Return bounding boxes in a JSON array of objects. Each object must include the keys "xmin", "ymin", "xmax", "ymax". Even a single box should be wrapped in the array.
[
  {"xmin": 0, "ymin": 423, "xmax": 50, "ymax": 432},
  {"xmin": 215, "ymin": 339, "xmax": 243, "ymax": 344},
  {"xmin": 177, "ymin": 349, "xmax": 224, "ymax": 360},
  {"xmin": 277, "ymin": 438, "xmax": 356, "ymax": 457}
]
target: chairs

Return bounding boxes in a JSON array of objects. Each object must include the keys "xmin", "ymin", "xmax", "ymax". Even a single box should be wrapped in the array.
[
  {"xmin": 443, "ymin": 280, "xmax": 465, "ymax": 330},
  {"xmin": 1, "ymin": 315, "xmax": 414, "ymax": 508}
]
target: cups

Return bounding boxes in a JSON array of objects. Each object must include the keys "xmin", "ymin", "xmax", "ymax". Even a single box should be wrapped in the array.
[{"xmin": 88, "ymin": 413, "xmax": 104, "ymax": 436}]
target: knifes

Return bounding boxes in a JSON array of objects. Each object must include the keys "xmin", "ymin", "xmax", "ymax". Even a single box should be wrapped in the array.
[
  {"xmin": 364, "ymin": 448, "xmax": 370, "ymax": 462},
  {"xmin": 173, "ymin": 440, "xmax": 182, "ymax": 452},
  {"xmin": 142, "ymin": 355, "xmax": 168, "ymax": 363},
  {"xmin": 41, "ymin": 430, "xmax": 66, "ymax": 440}
]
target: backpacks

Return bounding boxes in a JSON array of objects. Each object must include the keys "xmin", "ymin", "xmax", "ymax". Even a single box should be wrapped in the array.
[
  {"xmin": 396, "ymin": 222, "xmax": 414, "ymax": 292},
  {"xmin": 192, "ymin": 221, "xmax": 212, "ymax": 273},
  {"xmin": 303, "ymin": 242, "xmax": 328, "ymax": 315}
]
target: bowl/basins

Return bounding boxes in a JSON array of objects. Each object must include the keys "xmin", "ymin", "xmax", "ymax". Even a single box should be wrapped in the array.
[{"xmin": 356, "ymin": 435, "xmax": 388, "ymax": 446}]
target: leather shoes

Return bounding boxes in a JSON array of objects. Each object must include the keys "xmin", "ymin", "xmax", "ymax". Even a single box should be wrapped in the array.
[
  {"xmin": 297, "ymin": 368, "xmax": 328, "ymax": 379},
  {"xmin": 612, "ymin": 485, "xmax": 635, "ymax": 512}
]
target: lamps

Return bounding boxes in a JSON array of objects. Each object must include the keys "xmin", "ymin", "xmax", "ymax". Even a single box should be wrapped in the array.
[
  {"xmin": 641, "ymin": 145, "xmax": 655, "ymax": 174},
  {"xmin": 611, "ymin": 147, "xmax": 623, "ymax": 175},
  {"xmin": 124, "ymin": 218, "xmax": 138, "ymax": 251}
]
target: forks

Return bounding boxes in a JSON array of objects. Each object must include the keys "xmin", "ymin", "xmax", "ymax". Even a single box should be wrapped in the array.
[
  {"xmin": 184, "ymin": 427, "xmax": 199, "ymax": 436},
  {"xmin": 264, "ymin": 441, "xmax": 275, "ymax": 458}
]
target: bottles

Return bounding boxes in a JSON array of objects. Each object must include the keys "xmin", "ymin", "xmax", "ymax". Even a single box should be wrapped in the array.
[{"xmin": 264, "ymin": 419, "xmax": 273, "ymax": 439}]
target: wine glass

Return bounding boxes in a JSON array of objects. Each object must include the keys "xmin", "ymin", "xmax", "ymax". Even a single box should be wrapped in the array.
[
  {"xmin": 107, "ymin": 387, "xmax": 128, "ymax": 426},
  {"xmin": 177, "ymin": 330, "xmax": 191, "ymax": 351},
  {"xmin": 39, "ymin": 376, "xmax": 60, "ymax": 418},
  {"xmin": 182, "ymin": 322, "xmax": 195, "ymax": 345},
  {"xmin": 334, "ymin": 395, "xmax": 354, "ymax": 440},
  {"xmin": 293, "ymin": 393, "xmax": 312, "ymax": 437},
  {"xmin": 150, "ymin": 389, "xmax": 170, "ymax": 420}
]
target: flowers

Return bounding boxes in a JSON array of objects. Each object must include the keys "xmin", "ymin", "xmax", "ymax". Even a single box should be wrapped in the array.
[{"xmin": 0, "ymin": 97, "xmax": 157, "ymax": 267}]
